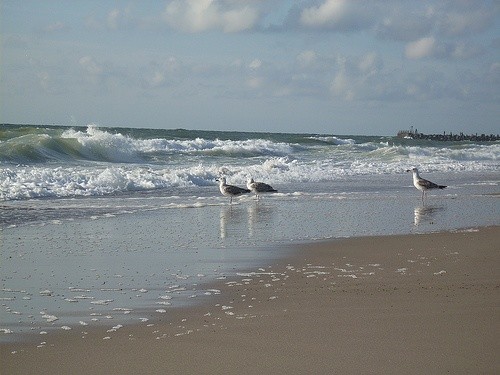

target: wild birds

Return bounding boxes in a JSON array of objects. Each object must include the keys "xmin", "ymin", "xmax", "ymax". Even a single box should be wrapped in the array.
[
  {"xmin": 247, "ymin": 177, "xmax": 279, "ymax": 200},
  {"xmin": 407, "ymin": 167, "xmax": 447, "ymax": 197},
  {"xmin": 215, "ymin": 177, "xmax": 251, "ymax": 204}
]
